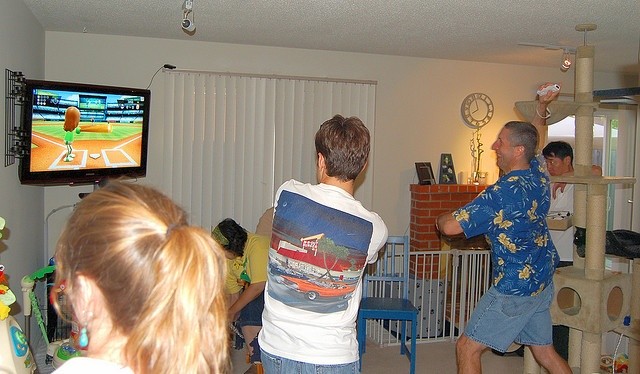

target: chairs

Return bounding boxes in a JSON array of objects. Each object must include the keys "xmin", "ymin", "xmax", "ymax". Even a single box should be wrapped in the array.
[{"xmin": 357, "ymin": 234, "xmax": 418, "ymax": 374}]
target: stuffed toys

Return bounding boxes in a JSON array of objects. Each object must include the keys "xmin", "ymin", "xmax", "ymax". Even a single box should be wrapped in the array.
[{"xmin": 0, "ymin": 279, "xmax": 18, "ymax": 314}]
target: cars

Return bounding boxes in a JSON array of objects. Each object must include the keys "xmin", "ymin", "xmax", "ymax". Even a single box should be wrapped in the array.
[{"xmin": 279, "ymin": 276, "xmax": 356, "ymax": 302}]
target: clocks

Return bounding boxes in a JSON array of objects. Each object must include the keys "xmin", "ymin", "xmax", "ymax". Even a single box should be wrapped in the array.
[{"xmin": 461, "ymin": 93, "xmax": 494, "ymax": 128}]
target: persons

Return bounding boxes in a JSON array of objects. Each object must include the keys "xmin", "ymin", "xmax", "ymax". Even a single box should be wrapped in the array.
[
  {"xmin": 211, "ymin": 217, "xmax": 271, "ymax": 374},
  {"xmin": 49, "ymin": 174, "xmax": 234, "ymax": 374},
  {"xmin": 436, "ymin": 82, "xmax": 572, "ymax": 374},
  {"xmin": 258, "ymin": 113, "xmax": 389, "ymax": 374},
  {"xmin": 542, "ymin": 141, "xmax": 602, "ymax": 362}
]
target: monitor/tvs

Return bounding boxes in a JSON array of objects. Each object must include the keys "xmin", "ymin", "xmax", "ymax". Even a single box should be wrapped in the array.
[{"xmin": 19, "ymin": 79, "xmax": 150, "ymax": 184}]
